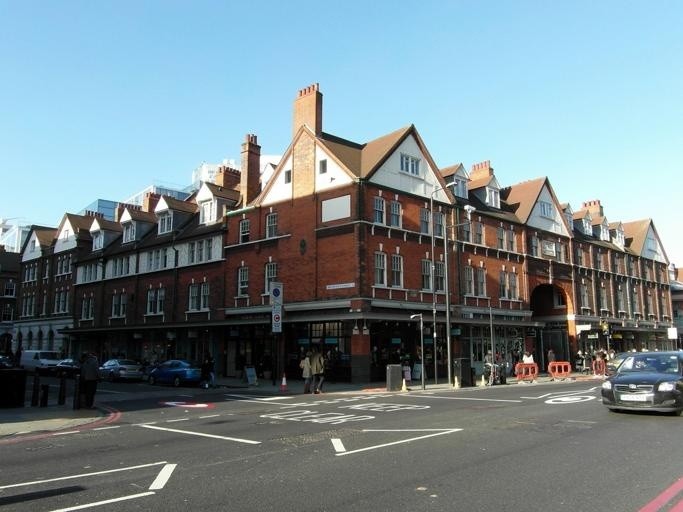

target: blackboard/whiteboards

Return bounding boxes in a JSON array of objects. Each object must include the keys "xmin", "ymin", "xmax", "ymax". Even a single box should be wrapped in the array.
[{"xmin": 246, "ymin": 366, "xmax": 256, "ymax": 384}]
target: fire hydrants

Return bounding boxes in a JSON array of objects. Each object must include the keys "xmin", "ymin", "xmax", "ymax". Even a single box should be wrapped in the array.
[{"xmin": 38, "ymin": 383, "xmax": 51, "ymax": 407}]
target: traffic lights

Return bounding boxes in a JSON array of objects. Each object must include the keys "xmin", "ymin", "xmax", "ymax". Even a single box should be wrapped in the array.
[{"xmin": 601, "ymin": 319, "xmax": 609, "ymax": 336}]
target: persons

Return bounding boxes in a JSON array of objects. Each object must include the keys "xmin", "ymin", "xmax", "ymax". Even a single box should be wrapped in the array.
[
  {"xmin": 80, "ymin": 352, "xmax": 101, "ymax": 410},
  {"xmin": 299, "ymin": 350, "xmax": 312, "ymax": 393},
  {"xmin": 208, "ymin": 357, "xmax": 217, "ymax": 389},
  {"xmin": 192, "ymin": 359, "xmax": 209, "ymax": 388},
  {"xmin": 310, "ymin": 346, "xmax": 324, "ymax": 394},
  {"xmin": 482, "ymin": 345, "xmax": 616, "ymax": 382}
]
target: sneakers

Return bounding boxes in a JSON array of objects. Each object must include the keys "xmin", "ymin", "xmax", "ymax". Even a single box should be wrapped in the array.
[{"xmin": 303, "ymin": 388, "xmax": 323, "ymax": 394}]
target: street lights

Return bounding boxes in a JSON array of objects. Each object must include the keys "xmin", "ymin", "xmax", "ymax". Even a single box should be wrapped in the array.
[{"xmin": 428, "ymin": 180, "xmax": 459, "ymax": 386}]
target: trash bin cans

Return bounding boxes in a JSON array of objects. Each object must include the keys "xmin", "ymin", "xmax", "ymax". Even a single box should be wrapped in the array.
[
  {"xmin": 386, "ymin": 364, "xmax": 402, "ymax": 390},
  {"xmin": 453, "ymin": 358, "xmax": 471, "ymax": 387}
]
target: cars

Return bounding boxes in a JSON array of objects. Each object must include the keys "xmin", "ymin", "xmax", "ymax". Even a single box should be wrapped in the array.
[
  {"xmin": 147, "ymin": 360, "xmax": 202, "ymax": 389},
  {"xmin": 0, "ymin": 348, "xmax": 144, "ymax": 385},
  {"xmin": 603, "ymin": 350, "xmax": 671, "ymax": 381},
  {"xmin": 600, "ymin": 351, "xmax": 682, "ymax": 417}
]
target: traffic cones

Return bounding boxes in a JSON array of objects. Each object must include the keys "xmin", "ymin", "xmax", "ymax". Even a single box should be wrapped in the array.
[
  {"xmin": 479, "ymin": 375, "xmax": 488, "ymax": 389},
  {"xmin": 452, "ymin": 376, "xmax": 460, "ymax": 390},
  {"xmin": 399, "ymin": 378, "xmax": 409, "ymax": 392},
  {"xmin": 279, "ymin": 372, "xmax": 289, "ymax": 393}
]
